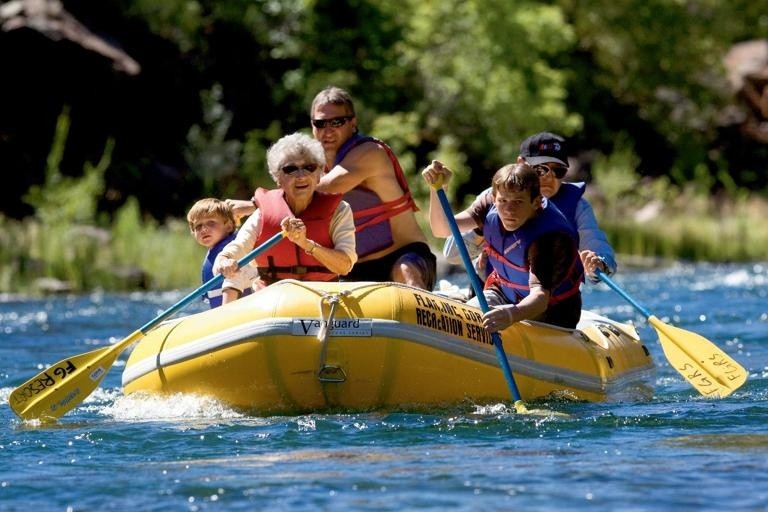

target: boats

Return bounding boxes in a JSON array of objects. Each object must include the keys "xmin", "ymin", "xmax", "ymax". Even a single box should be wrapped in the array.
[{"xmin": 122, "ymin": 280, "xmax": 654, "ymax": 420}]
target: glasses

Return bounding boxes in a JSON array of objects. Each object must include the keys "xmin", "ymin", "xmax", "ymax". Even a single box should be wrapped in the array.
[
  {"xmin": 526, "ymin": 164, "xmax": 567, "ymax": 179},
  {"xmin": 311, "ymin": 115, "xmax": 355, "ymax": 129},
  {"xmin": 282, "ymin": 164, "xmax": 318, "ymax": 174}
]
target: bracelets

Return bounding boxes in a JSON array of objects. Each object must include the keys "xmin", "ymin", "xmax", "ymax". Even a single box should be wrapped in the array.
[
  {"xmin": 596, "ymin": 255, "xmax": 607, "ymax": 273},
  {"xmin": 305, "ymin": 240, "xmax": 318, "ymax": 257}
]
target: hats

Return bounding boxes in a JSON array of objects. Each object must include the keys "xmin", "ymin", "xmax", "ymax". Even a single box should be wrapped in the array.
[{"xmin": 520, "ymin": 132, "xmax": 569, "ymax": 167}]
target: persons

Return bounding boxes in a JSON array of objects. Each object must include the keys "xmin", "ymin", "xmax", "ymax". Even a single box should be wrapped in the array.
[
  {"xmin": 208, "ymin": 132, "xmax": 358, "ymax": 288},
  {"xmin": 306, "ymin": 86, "xmax": 436, "ymax": 293},
  {"xmin": 187, "ymin": 196, "xmax": 254, "ymax": 310},
  {"xmin": 421, "ymin": 130, "xmax": 618, "ymax": 311},
  {"xmin": 421, "ymin": 159, "xmax": 585, "ymax": 333}
]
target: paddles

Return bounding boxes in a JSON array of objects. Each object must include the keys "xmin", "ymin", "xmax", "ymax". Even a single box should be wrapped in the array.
[
  {"xmin": 9, "ymin": 229, "xmax": 289, "ymax": 420},
  {"xmin": 595, "ymin": 269, "xmax": 748, "ymax": 400}
]
